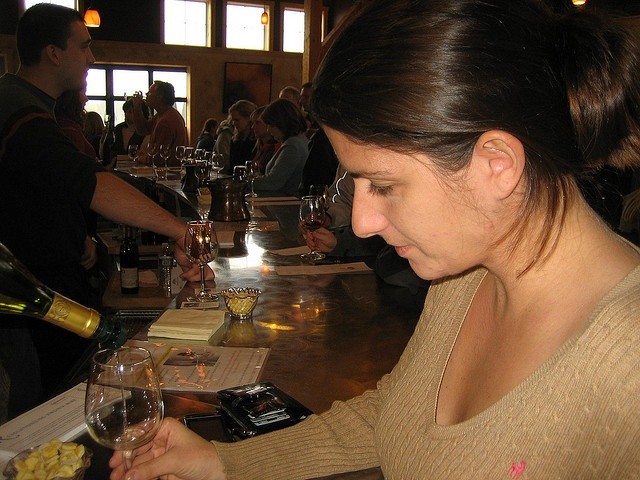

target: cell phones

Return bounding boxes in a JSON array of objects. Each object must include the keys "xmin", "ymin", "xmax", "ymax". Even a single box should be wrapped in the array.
[{"xmin": 182, "ymin": 411, "xmax": 237, "ymax": 445}]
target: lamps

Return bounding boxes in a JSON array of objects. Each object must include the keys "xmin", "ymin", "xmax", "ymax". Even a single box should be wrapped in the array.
[
  {"xmin": 261, "ymin": 0, "xmax": 268, "ymax": 25},
  {"xmin": 83, "ymin": 0, "xmax": 101, "ymax": 27}
]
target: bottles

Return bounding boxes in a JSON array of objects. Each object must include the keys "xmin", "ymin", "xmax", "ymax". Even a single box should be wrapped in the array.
[{"xmin": 0, "ymin": 238, "xmax": 120, "ymax": 346}]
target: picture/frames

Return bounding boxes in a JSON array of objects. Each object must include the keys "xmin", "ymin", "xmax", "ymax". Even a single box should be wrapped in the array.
[{"xmin": 222, "ymin": 62, "xmax": 272, "ymax": 114}]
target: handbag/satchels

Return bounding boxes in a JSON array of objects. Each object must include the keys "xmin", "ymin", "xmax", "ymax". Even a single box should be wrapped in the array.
[{"xmin": 214, "ymin": 381, "xmax": 316, "ymax": 442}]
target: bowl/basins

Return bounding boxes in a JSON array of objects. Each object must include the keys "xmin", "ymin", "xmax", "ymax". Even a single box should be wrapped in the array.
[
  {"xmin": 1, "ymin": 439, "xmax": 95, "ymax": 476},
  {"xmin": 220, "ymin": 285, "xmax": 264, "ymax": 322}
]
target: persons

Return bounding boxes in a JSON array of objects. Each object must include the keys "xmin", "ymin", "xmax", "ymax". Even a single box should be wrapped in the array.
[
  {"xmin": 278, "ymin": 86, "xmax": 309, "ymax": 127},
  {"xmin": 225, "ymin": 100, "xmax": 257, "ymax": 177},
  {"xmin": 246, "ymin": 99, "xmax": 309, "ymax": 192},
  {"xmin": 133, "ymin": 81, "xmax": 188, "ymax": 167},
  {"xmin": 106, "ymin": 0, "xmax": 640, "ymax": 479},
  {"xmin": 1, "ymin": 3, "xmax": 217, "ymax": 403},
  {"xmin": 300, "ymin": 163, "xmax": 354, "ymax": 231},
  {"xmin": 212, "ymin": 113, "xmax": 236, "ymax": 170},
  {"xmin": 300, "ymin": 82, "xmax": 338, "ymax": 196},
  {"xmin": 54, "ymin": 81, "xmax": 98, "ymax": 164},
  {"xmin": 302, "ymin": 227, "xmax": 422, "ymax": 294},
  {"xmin": 250, "ymin": 104, "xmax": 282, "ymax": 174},
  {"xmin": 195, "ymin": 118, "xmax": 218, "ymax": 153},
  {"xmin": 83, "ymin": 112, "xmax": 105, "ymax": 157},
  {"xmin": 113, "ymin": 98, "xmax": 155, "ymax": 155}
]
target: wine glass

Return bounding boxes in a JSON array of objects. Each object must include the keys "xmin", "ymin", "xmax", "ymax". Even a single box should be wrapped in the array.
[
  {"xmin": 310, "ymin": 185, "xmax": 332, "ymax": 212},
  {"xmin": 182, "ymin": 146, "xmax": 202, "ymax": 160},
  {"xmin": 193, "ymin": 148, "xmax": 206, "ymax": 162},
  {"xmin": 145, "ymin": 139, "xmax": 160, "ymax": 171},
  {"xmin": 194, "ymin": 159, "xmax": 212, "ymax": 189},
  {"xmin": 211, "ymin": 153, "xmax": 227, "ymax": 180},
  {"xmin": 203, "ymin": 150, "xmax": 216, "ymax": 163},
  {"xmin": 298, "ymin": 191, "xmax": 330, "ymax": 265},
  {"xmin": 232, "ymin": 164, "xmax": 250, "ymax": 207},
  {"xmin": 158, "ymin": 141, "xmax": 174, "ymax": 173},
  {"xmin": 81, "ymin": 343, "xmax": 167, "ymax": 474},
  {"xmin": 156, "ymin": 240, "xmax": 179, "ymax": 303},
  {"xmin": 128, "ymin": 142, "xmax": 140, "ymax": 171},
  {"xmin": 185, "ymin": 219, "xmax": 221, "ymax": 305},
  {"xmin": 245, "ymin": 159, "xmax": 261, "ymax": 200},
  {"xmin": 174, "ymin": 146, "xmax": 186, "ymax": 173}
]
